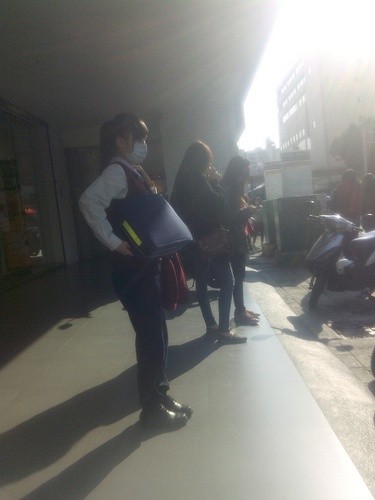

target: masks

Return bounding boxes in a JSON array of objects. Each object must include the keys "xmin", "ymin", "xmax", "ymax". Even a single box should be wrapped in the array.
[{"xmin": 124, "ymin": 143, "xmax": 147, "ymax": 164}]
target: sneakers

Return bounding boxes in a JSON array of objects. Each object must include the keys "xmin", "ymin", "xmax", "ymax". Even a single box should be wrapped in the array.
[
  {"xmin": 246, "ymin": 310, "xmax": 260, "ymax": 317},
  {"xmin": 139, "ymin": 405, "xmax": 188, "ymax": 428},
  {"xmin": 165, "ymin": 401, "xmax": 192, "ymax": 420},
  {"xmin": 235, "ymin": 312, "xmax": 259, "ymax": 326},
  {"xmin": 215, "ymin": 331, "xmax": 248, "ymax": 344},
  {"xmin": 206, "ymin": 324, "xmax": 233, "ymax": 335}
]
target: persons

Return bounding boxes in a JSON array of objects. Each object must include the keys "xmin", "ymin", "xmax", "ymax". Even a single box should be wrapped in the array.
[
  {"xmin": 330, "ymin": 169, "xmax": 375, "ymax": 227},
  {"xmin": 251, "ymin": 196, "xmax": 267, "ymax": 247},
  {"xmin": 78, "ymin": 113, "xmax": 194, "ymax": 426},
  {"xmin": 222, "ymin": 155, "xmax": 261, "ymax": 323},
  {"xmin": 168, "ymin": 141, "xmax": 247, "ymax": 343}
]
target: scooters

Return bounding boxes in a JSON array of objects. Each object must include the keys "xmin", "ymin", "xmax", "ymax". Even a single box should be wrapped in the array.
[{"xmin": 305, "ymin": 213, "xmax": 375, "ymax": 309}]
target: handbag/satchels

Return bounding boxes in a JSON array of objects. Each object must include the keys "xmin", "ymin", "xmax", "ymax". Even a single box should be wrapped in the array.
[
  {"xmin": 97, "ymin": 161, "xmax": 194, "ymax": 258},
  {"xmin": 162, "ymin": 252, "xmax": 188, "ymax": 309}
]
